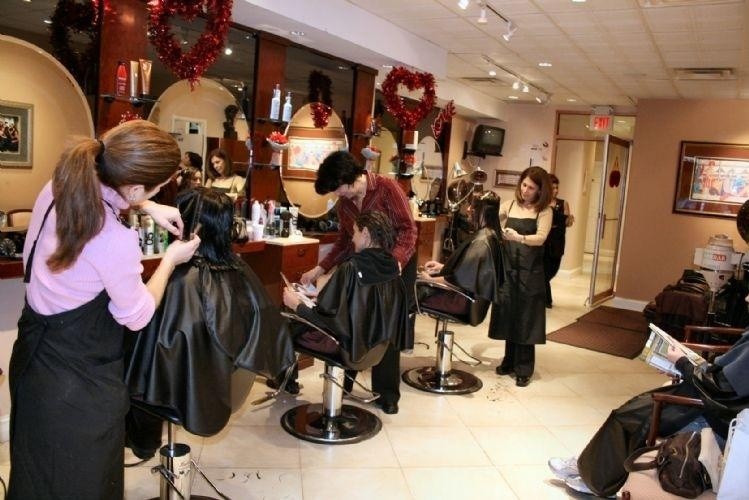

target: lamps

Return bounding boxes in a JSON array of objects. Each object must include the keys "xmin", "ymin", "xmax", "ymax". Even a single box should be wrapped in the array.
[
  {"xmin": 477, "ymin": 54, "xmax": 550, "ymax": 106},
  {"xmin": 467, "ymin": 166, "xmax": 489, "ymax": 185},
  {"xmin": 448, "ymin": 162, "xmax": 468, "ymax": 180},
  {"xmin": 457, "ymin": 0, "xmax": 518, "ymax": 43}
]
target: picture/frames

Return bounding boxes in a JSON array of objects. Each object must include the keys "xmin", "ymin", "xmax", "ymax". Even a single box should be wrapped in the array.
[
  {"xmin": 0, "ymin": 100, "xmax": 34, "ymax": 168},
  {"xmin": 671, "ymin": 141, "xmax": 749, "ymax": 219},
  {"xmin": 282, "ymin": 127, "xmax": 346, "ymax": 180}
]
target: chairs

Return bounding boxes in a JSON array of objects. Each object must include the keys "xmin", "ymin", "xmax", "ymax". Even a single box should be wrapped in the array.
[
  {"xmin": 280, "ymin": 277, "xmax": 406, "ymax": 446},
  {"xmin": 126, "ymin": 325, "xmax": 259, "ymax": 500},
  {"xmin": 645, "ymin": 337, "xmax": 737, "ymax": 447},
  {"xmin": 672, "ymin": 324, "xmax": 748, "ymax": 353},
  {"xmin": 401, "ymin": 255, "xmax": 493, "ymax": 395}
]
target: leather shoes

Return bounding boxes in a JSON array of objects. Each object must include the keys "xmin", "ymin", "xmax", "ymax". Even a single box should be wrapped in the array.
[
  {"xmin": 516, "ymin": 372, "xmax": 531, "ymax": 387},
  {"xmin": 496, "ymin": 366, "xmax": 515, "ymax": 374},
  {"xmin": 373, "ymin": 390, "xmax": 399, "ymax": 414},
  {"xmin": 267, "ymin": 380, "xmax": 300, "ymax": 395}
]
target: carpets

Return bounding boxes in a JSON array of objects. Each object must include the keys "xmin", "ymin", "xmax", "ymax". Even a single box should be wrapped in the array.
[{"xmin": 545, "ymin": 303, "xmax": 653, "ymax": 360}]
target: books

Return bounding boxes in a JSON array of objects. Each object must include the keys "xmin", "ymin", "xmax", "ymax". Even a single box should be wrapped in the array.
[
  {"xmin": 637, "ymin": 323, "xmax": 712, "ymax": 382},
  {"xmin": 290, "ymin": 282, "xmax": 320, "ymax": 309}
]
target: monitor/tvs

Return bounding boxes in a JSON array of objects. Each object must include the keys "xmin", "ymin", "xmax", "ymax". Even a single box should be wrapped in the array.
[{"xmin": 471, "ymin": 124, "xmax": 506, "ymax": 155}]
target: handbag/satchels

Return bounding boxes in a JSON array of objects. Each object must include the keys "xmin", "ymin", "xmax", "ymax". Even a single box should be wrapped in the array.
[{"xmin": 623, "ymin": 430, "xmax": 715, "ymax": 499}]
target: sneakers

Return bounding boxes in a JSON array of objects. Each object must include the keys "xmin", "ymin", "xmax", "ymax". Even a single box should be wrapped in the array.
[
  {"xmin": 546, "ymin": 455, "xmax": 581, "ymax": 479},
  {"xmin": 565, "ymin": 473, "xmax": 617, "ymax": 499}
]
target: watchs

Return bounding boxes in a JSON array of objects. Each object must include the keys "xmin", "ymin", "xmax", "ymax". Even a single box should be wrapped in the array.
[{"xmin": 521, "ymin": 236, "xmax": 528, "ymax": 245}]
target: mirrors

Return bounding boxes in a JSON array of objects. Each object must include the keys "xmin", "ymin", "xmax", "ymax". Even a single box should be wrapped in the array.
[
  {"xmin": 411, "ymin": 136, "xmax": 444, "ymax": 203},
  {"xmin": 278, "ymin": 101, "xmax": 351, "ymax": 220},
  {"xmin": 0, "ymin": 33, "xmax": 96, "ymax": 241},
  {"xmin": 171, "ymin": 114, "xmax": 208, "ymax": 188},
  {"xmin": 364, "ymin": 126, "xmax": 400, "ymax": 182},
  {"xmin": 145, "ymin": 74, "xmax": 252, "ymax": 202}
]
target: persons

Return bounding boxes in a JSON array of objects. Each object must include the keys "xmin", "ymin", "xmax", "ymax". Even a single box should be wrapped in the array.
[
  {"xmin": 545, "ymin": 173, "xmax": 574, "ymax": 308},
  {"xmin": 547, "ymin": 197, "xmax": 749, "ymax": 500},
  {"xmin": 6, "ymin": 119, "xmax": 202, "ymax": 500},
  {"xmin": 419, "ymin": 190, "xmax": 503, "ymax": 314},
  {"xmin": 172, "ymin": 169, "xmax": 202, "ymax": 196},
  {"xmin": 205, "ymin": 150, "xmax": 245, "ymax": 204},
  {"xmin": 497, "ymin": 167, "xmax": 554, "ymax": 385},
  {"xmin": 299, "ymin": 150, "xmax": 418, "ymax": 414},
  {"xmin": 177, "ymin": 152, "xmax": 200, "ymax": 168},
  {"xmin": 267, "ymin": 210, "xmax": 405, "ymax": 394},
  {"xmin": 124, "ymin": 186, "xmax": 274, "ymax": 460}
]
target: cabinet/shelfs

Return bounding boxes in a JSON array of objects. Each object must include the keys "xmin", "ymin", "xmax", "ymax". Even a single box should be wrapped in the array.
[
  {"xmin": 414, "ymin": 217, "xmax": 435, "ymax": 270},
  {"xmin": 140, "ymin": 239, "xmax": 319, "ymax": 371}
]
